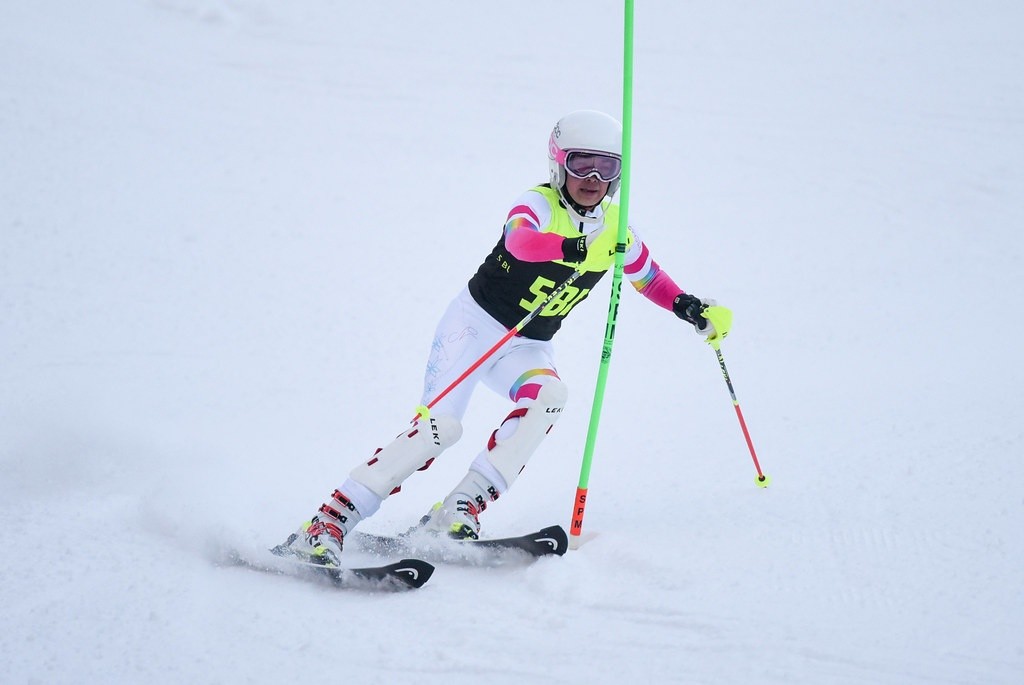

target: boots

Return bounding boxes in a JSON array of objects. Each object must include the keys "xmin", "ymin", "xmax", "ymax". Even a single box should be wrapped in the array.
[
  {"xmin": 287, "ymin": 477, "xmax": 382, "ymax": 567},
  {"xmin": 420, "ymin": 455, "xmax": 508, "ymax": 540}
]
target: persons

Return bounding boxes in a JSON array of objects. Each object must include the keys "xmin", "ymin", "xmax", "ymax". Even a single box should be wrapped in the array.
[{"xmin": 276, "ymin": 111, "xmax": 722, "ymax": 566}]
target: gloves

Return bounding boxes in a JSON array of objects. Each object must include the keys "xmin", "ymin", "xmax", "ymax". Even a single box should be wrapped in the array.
[
  {"xmin": 561, "ymin": 226, "xmax": 605, "ymax": 262},
  {"xmin": 673, "ymin": 293, "xmax": 718, "ymax": 336}
]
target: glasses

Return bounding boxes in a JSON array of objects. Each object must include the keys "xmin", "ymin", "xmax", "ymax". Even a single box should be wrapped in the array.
[{"xmin": 564, "ymin": 150, "xmax": 623, "ymax": 183}]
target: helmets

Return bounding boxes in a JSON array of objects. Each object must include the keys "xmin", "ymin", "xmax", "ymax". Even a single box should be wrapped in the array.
[{"xmin": 549, "ymin": 110, "xmax": 624, "ymax": 198}]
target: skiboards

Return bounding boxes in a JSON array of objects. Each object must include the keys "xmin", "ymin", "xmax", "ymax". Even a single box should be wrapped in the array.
[
  {"xmin": 227, "ymin": 544, "xmax": 436, "ymax": 594},
  {"xmin": 353, "ymin": 524, "xmax": 569, "ymax": 570}
]
